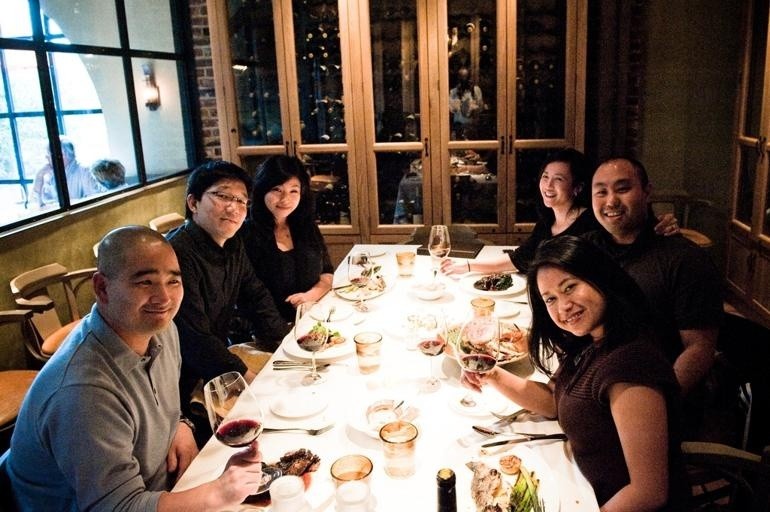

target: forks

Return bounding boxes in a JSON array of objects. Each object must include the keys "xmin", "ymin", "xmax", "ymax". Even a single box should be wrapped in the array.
[
  {"xmin": 491, "ymin": 410, "xmax": 521, "ymax": 425},
  {"xmin": 260, "ymin": 423, "xmax": 334, "ymax": 436}
]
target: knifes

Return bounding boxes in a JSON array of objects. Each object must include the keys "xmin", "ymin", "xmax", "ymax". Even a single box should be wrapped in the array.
[
  {"xmin": 272, "ymin": 358, "xmax": 333, "ymax": 366},
  {"xmin": 482, "ymin": 432, "xmax": 567, "ymax": 448}
]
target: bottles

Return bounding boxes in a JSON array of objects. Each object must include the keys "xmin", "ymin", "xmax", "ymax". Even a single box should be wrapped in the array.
[
  {"xmin": 233, "ymin": 0, "xmax": 567, "ymax": 145},
  {"xmin": 318, "ymin": 181, "xmax": 350, "ymax": 226},
  {"xmin": 436, "ymin": 468, "xmax": 459, "ymax": 512},
  {"xmin": 398, "ymin": 184, "xmax": 424, "ymax": 224},
  {"xmin": 406, "ymin": 314, "xmax": 417, "ymax": 353}
]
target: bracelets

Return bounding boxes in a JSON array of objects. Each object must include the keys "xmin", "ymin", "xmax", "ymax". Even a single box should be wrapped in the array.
[
  {"xmin": 179, "ymin": 415, "xmax": 198, "ymax": 432},
  {"xmin": 467, "ymin": 259, "xmax": 470, "ymax": 272}
]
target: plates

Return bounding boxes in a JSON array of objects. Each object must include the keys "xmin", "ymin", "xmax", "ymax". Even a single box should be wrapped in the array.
[
  {"xmin": 492, "ymin": 299, "xmax": 521, "ymax": 318},
  {"xmin": 335, "ymin": 281, "xmax": 385, "ymax": 300},
  {"xmin": 269, "ymin": 386, "xmax": 330, "ymax": 417},
  {"xmin": 352, "ymin": 407, "xmax": 418, "ymax": 440},
  {"xmin": 415, "ymin": 285, "xmax": 445, "ymax": 301},
  {"xmin": 285, "ymin": 329, "xmax": 355, "ymax": 361},
  {"xmin": 310, "ymin": 303, "xmax": 352, "ymax": 322},
  {"xmin": 465, "ymin": 273, "xmax": 528, "ymax": 295},
  {"xmin": 369, "ymin": 247, "xmax": 387, "ymax": 257}
]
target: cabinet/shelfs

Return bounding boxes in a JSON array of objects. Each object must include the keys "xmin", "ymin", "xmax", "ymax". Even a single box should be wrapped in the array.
[
  {"xmin": 720, "ymin": 0, "xmax": 770, "ymax": 331},
  {"xmin": 206, "ymin": 0, "xmax": 588, "ymax": 274}
]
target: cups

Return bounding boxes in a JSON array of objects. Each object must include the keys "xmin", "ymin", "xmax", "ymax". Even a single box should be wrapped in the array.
[
  {"xmin": 366, "ymin": 399, "xmax": 404, "ymax": 430},
  {"xmin": 354, "ymin": 330, "xmax": 384, "ymax": 373},
  {"xmin": 470, "ymin": 298, "xmax": 496, "ymax": 317},
  {"xmin": 396, "ymin": 249, "xmax": 414, "ymax": 277},
  {"xmin": 380, "ymin": 420, "xmax": 419, "ymax": 480},
  {"xmin": 269, "ymin": 475, "xmax": 308, "ymax": 512},
  {"xmin": 329, "ymin": 454, "xmax": 375, "ymax": 504}
]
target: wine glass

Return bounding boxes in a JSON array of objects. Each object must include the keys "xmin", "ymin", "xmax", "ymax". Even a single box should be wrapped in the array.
[
  {"xmin": 203, "ymin": 371, "xmax": 282, "ymax": 496},
  {"xmin": 349, "ymin": 250, "xmax": 375, "ymax": 311},
  {"xmin": 416, "ymin": 311, "xmax": 448, "ymax": 391},
  {"xmin": 457, "ymin": 307, "xmax": 503, "ymax": 409},
  {"xmin": 295, "ymin": 301, "xmax": 328, "ymax": 387},
  {"xmin": 427, "ymin": 224, "xmax": 451, "ymax": 286}
]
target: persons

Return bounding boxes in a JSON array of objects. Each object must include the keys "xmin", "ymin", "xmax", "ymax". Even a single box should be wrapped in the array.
[
  {"xmin": 449, "ymin": 67, "xmax": 483, "ymax": 115},
  {"xmin": 459, "ymin": 236, "xmax": 687, "ymax": 512},
  {"xmin": 164, "ymin": 160, "xmax": 294, "ymax": 409},
  {"xmin": 583, "ymin": 152, "xmax": 726, "ymax": 399},
  {"xmin": 27, "ymin": 135, "xmax": 108, "ymax": 209},
  {"xmin": 5, "ymin": 225, "xmax": 262, "ymax": 512},
  {"xmin": 230, "ymin": 153, "xmax": 334, "ymax": 342},
  {"xmin": 440, "ymin": 149, "xmax": 681, "ymax": 277}
]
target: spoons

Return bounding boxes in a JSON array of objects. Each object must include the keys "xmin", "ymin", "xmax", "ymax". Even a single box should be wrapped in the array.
[
  {"xmin": 274, "ymin": 362, "xmax": 331, "ymax": 373},
  {"xmin": 472, "ymin": 425, "xmax": 547, "ymax": 438}
]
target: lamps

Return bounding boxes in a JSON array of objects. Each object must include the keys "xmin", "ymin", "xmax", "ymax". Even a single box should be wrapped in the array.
[{"xmin": 143, "ymin": 75, "xmax": 160, "ymax": 110}]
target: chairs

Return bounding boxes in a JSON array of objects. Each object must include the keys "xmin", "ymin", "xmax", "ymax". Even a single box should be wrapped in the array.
[
  {"xmin": 679, "ymin": 441, "xmax": 770, "ymax": 512},
  {"xmin": 0, "ymin": 212, "xmax": 187, "ymax": 446},
  {"xmin": 649, "ymin": 195, "xmax": 714, "ymax": 228}
]
